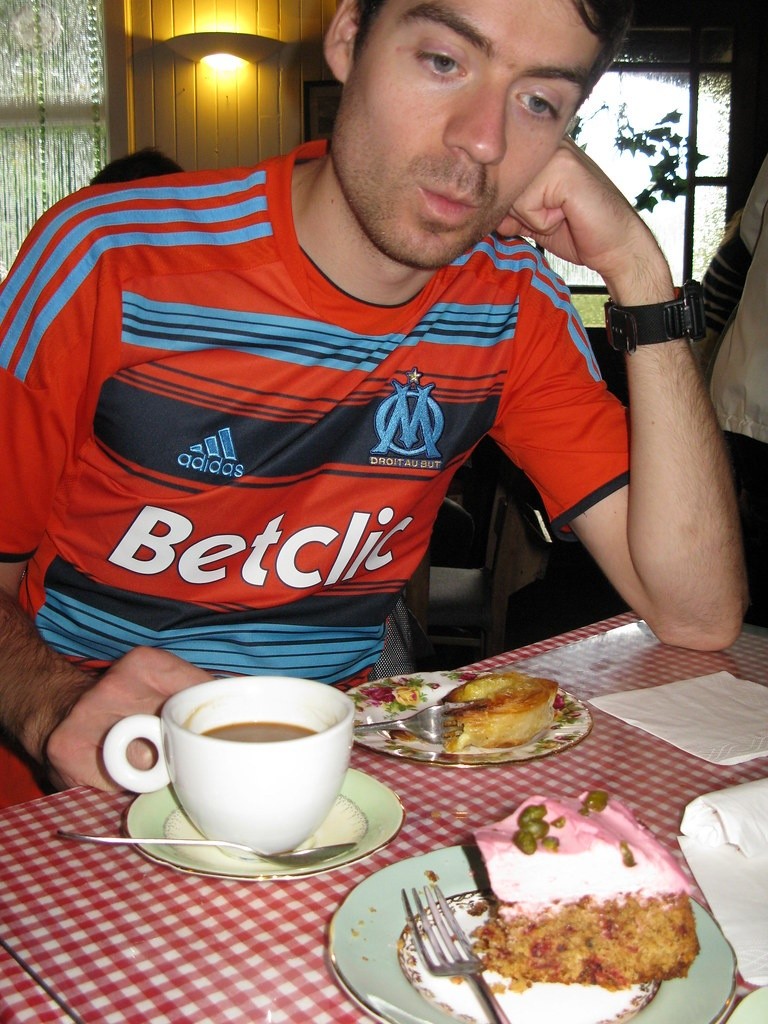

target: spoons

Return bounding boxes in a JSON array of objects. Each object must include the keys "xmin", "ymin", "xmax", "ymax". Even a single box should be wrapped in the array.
[{"xmin": 56, "ymin": 830, "xmax": 358, "ymax": 868}]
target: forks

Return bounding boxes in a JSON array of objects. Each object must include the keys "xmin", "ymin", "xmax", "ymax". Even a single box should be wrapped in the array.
[
  {"xmin": 353, "ymin": 702, "xmax": 472, "ymax": 745},
  {"xmin": 400, "ymin": 885, "xmax": 513, "ymax": 1024}
]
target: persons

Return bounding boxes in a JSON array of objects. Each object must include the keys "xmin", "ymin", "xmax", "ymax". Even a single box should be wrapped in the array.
[
  {"xmin": 700, "ymin": 152, "xmax": 767, "ymax": 628},
  {"xmin": 90, "ymin": 144, "xmax": 184, "ymax": 186},
  {"xmin": 3, "ymin": 1, "xmax": 750, "ymax": 806}
]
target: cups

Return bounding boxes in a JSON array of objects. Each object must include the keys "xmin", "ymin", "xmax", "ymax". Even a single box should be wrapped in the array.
[{"xmin": 101, "ymin": 674, "xmax": 359, "ymax": 860}]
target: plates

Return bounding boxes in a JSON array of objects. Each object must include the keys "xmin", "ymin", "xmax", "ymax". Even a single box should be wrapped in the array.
[
  {"xmin": 344, "ymin": 670, "xmax": 594, "ymax": 767},
  {"xmin": 328, "ymin": 839, "xmax": 740, "ymax": 1024},
  {"xmin": 121, "ymin": 767, "xmax": 406, "ymax": 880}
]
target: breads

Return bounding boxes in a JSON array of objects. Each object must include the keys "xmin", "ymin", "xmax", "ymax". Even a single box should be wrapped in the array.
[{"xmin": 434, "ymin": 673, "xmax": 559, "ymax": 750}]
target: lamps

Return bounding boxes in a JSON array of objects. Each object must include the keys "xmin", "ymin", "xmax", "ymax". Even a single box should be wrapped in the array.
[{"xmin": 165, "ymin": 26, "xmax": 282, "ymax": 72}]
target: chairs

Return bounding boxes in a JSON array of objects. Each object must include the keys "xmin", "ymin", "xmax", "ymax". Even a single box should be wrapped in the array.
[{"xmin": 396, "ymin": 446, "xmax": 529, "ymax": 661}]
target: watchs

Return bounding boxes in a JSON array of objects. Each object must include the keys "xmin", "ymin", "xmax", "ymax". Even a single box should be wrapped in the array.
[{"xmin": 601, "ymin": 276, "xmax": 709, "ymax": 358}]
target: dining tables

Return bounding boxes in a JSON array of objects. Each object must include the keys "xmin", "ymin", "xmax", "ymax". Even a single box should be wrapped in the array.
[{"xmin": 2, "ymin": 608, "xmax": 767, "ymax": 1023}]
